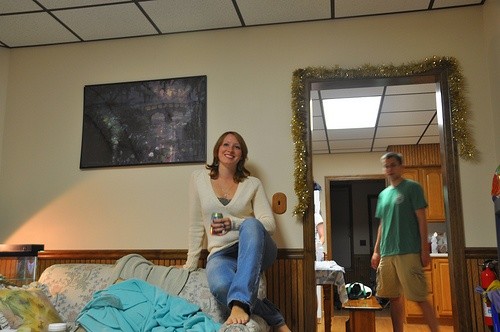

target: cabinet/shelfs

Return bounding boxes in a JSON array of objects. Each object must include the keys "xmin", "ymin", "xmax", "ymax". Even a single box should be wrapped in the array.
[
  {"xmin": 401, "ymin": 168, "xmax": 446, "ymax": 222},
  {"xmin": 403, "ymin": 257, "xmax": 455, "ymax": 326}
]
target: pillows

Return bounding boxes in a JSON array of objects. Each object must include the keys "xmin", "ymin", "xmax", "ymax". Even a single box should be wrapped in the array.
[{"xmin": 0, "ymin": 283, "xmax": 67, "ymax": 332}]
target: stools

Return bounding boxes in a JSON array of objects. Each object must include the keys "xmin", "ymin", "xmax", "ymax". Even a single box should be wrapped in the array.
[{"xmin": 341, "ymin": 294, "xmax": 383, "ymax": 332}]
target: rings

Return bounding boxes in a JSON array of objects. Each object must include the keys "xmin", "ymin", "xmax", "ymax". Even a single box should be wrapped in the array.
[{"xmin": 222, "ymin": 223, "xmax": 225, "ymax": 226}]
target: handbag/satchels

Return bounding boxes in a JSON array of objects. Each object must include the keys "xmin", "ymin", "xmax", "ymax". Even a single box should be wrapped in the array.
[{"xmin": 345, "ymin": 282, "xmax": 372, "ymax": 299}]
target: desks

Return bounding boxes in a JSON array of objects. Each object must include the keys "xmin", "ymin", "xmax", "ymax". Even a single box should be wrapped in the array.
[{"xmin": 315, "ymin": 261, "xmax": 348, "ymax": 332}]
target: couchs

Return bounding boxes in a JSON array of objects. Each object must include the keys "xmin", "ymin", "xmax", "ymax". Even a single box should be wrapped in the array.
[{"xmin": 6, "ymin": 263, "xmax": 272, "ymax": 332}]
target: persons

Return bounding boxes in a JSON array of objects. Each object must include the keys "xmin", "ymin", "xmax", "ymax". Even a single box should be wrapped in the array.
[
  {"xmin": 183, "ymin": 132, "xmax": 291, "ymax": 332},
  {"xmin": 371, "ymin": 153, "xmax": 438, "ymax": 332},
  {"xmin": 314, "ymin": 212, "xmax": 325, "ymax": 324},
  {"xmin": 474, "ymin": 259, "xmax": 500, "ymax": 332}
]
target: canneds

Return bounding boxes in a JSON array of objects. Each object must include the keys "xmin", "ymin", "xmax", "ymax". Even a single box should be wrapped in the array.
[{"xmin": 210, "ymin": 212, "xmax": 223, "ymax": 236}]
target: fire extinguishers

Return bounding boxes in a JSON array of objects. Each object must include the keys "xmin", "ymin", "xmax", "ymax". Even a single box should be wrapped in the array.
[{"xmin": 479, "ymin": 259, "xmax": 498, "ymax": 327}]
